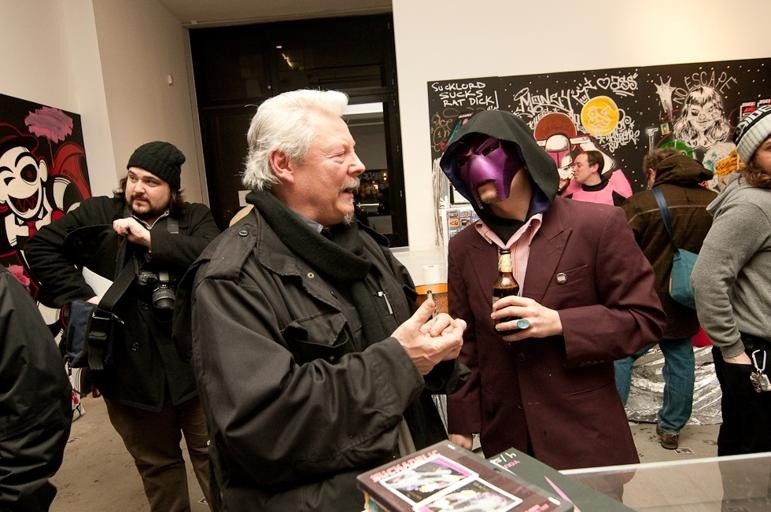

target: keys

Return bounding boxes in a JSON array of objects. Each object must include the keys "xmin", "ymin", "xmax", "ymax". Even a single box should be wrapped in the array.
[{"xmin": 750, "ymin": 350, "xmax": 770, "ymax": 392}]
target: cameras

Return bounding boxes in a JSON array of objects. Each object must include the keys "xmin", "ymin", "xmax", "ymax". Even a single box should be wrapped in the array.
[{"xmin": 138, "ymin": 271, "xmax": 178, "ymax": 321}]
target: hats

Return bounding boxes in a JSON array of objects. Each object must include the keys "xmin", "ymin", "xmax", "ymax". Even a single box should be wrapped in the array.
[
  {"xmin": 731, "ymin": 103, "xmax": 771, "ymax": 161},
  {"xmin": 127, "ymin": 141, "xmax": 185, "ymax": 191}
]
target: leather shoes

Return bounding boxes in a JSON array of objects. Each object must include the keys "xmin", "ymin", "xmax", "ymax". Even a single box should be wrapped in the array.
[{"xmin": 654, "ymin": 421, "xmax": 680, "ymax": 449}]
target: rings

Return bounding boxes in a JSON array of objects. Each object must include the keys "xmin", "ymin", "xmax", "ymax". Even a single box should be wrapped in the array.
[{"xmin": 517, "ymin": 318, "xmax": 529, "ymax": 328}]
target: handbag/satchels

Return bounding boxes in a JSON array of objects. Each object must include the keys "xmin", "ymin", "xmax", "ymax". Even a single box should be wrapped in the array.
[
  {"xmin": 61, "ymin": 220, "xmax": 142, "ymax": 372},
  {"xmin": 651, "ymin": 183, "xmax": 697, "ymax": 310}
]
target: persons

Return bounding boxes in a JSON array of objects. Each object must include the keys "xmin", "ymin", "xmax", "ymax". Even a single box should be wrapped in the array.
[
  {"xmin": 28, "ymin": 143, "xmax": 220, "ymax": 511},
  {"xmin": 614, "ymin": 149, "xmax": 720, "ymax": 450},
  {"xmin": 0, "ymin": 266, "xmax": 72, "ymax": 512},
  {"xmin": 438, "ymin": 111, "xmax": 671, "ymax": 499},
  {"xmin": 559, "ymin": 152, "xmax": 626, "ymax": 208},
  {"xmin": 189, "ymin": 90, "xmax": 464, "ymax": 512},
  {"xmin": 690, "ymin": 103, "xmax": 771, "ymax": 512}
]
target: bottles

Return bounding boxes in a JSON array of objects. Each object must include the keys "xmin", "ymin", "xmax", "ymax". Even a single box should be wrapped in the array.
[{"xmin": 491, "ymin": 248, "xmax": 520, "ymax": 335}]
